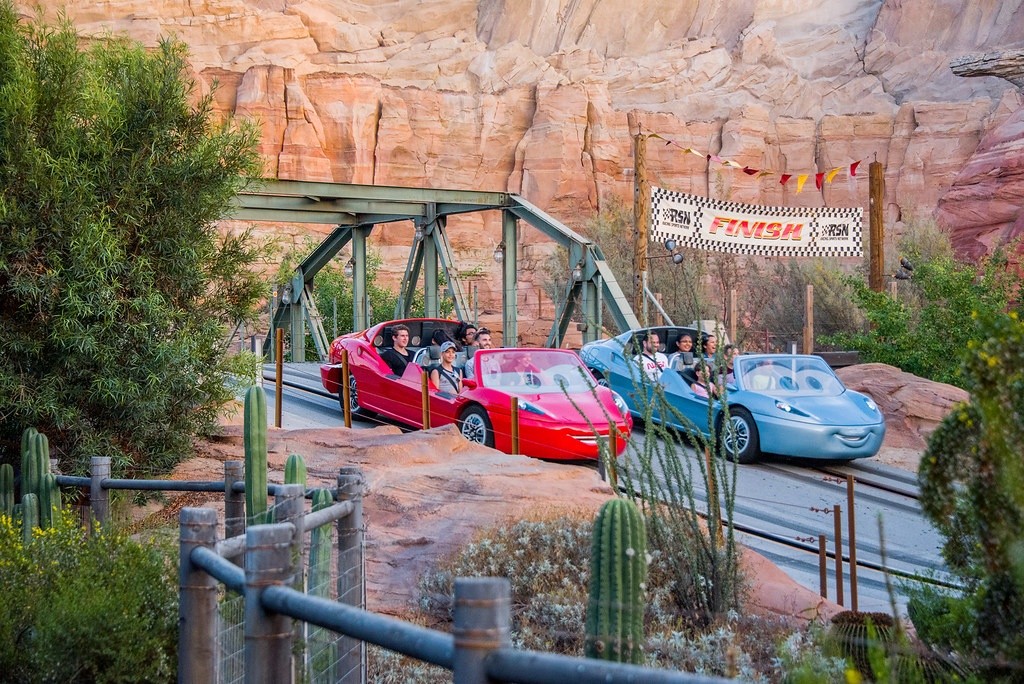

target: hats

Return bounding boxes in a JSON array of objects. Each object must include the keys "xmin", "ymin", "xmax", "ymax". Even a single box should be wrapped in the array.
[{"xmin": 440, "ymin": 341, "xmax": 457, "ymax": 352}]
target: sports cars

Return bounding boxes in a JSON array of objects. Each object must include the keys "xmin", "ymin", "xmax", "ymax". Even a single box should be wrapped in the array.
[
  {"xmin": 321, "ymin": 316, "xmax": 633, "ymax": 460},
  {"xmin": 577, "ymin": 325, "xmax": 887, "ymax": 464}
]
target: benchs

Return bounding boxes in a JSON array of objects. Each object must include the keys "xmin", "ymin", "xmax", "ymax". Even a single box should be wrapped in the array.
[
  {"xmin": 380, "ymin": 347, "xmax": 423, "ymax": 353},
  {"xmin": 669, "ymin": 354, "xmax": 716, "ymax": 371},
  {"xmin": 412, "ymin": 346, "xmax": 472, "ymax": 371}
]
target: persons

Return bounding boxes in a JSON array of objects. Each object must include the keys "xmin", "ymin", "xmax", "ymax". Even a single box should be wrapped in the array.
[
  {"xmin": 632, "ymin": 331, "xmax": 669, "ymax": 381},
  {"xmin": 466, "ymin": 326, "xmax": 502, "ymax": 388},
  {"xmin": 700, "ymin": 335, "xmax": 720, "ymax": 365},
  {"xmin": 430, "ymin": 340, "xmax": 464, "ymax": 395},
  {"xmin": 505, "ymin": 352, "xmax": 535, "ymax": 386},
  {"xmin": 459, "ymin": 324, "xmax": 478, "ymax": 346},
  {"xmin": 690, "ymin": 361, "xmax": 720, "ymax": 400},
  {"xmin": 431, "ymin": 330, "xmax": 447, "ymax": 349},
  {"xmin": 716, "ymin": 344, "xmax": 740, "ymax": 393},
  {"xmin": 667, "ymin": 333, "xmax": 698, "ymax": 372},
  {"xmin": 378, "ymin": 324, "xmax": 416, "ymax": 377}
]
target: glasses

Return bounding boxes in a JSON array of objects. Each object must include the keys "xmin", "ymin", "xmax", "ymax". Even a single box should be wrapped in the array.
[
  {"xmin": 680, "ymin": 341, "xmax": 692, "ymax": 344},
  {"xmin": 468, "ymin": 332, "xmax": 475, "ymax": 334}
]
